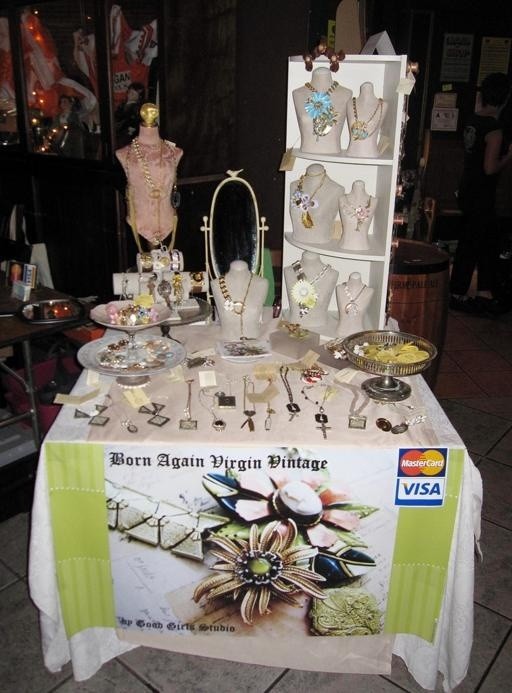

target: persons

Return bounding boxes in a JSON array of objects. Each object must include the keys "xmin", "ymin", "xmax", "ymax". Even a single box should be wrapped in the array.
[
  {"xmin": 45, "ymin": 94, "xmax": 86, "ymax": 160},
  {"xmin": 447, "ymin": 73, "xmax": 512, "ymax": 313},
  {"xmin": 114, "ymin": 83, "xmax": 145, "ymax": 145}
]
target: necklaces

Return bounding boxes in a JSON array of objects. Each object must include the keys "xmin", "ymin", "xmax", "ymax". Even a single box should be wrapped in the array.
[
  {"xmin": 219, "ymin": 271, "xmax": 254, "ymax": 335},
  {"xmin": 130, "ymin": 136, "xmax": 164, "ymax": 198},
  {"xmin": 292, "ymin": 260, "xmax": 330, "ymax": 318},
  {"xmin": 343, "ymin": 282, "xmax": 368, "ymax": 318},
  {"xmin": 351, "ymin": 94, "xmax": 384, "ymax": 141},
  {"xmin": 99, "ymin": 365, "xmax": 428, "ymax": 440},
  {"xmin": 294, "ymin": 169, "xmax": 329, "ymax": 230},
  {"xmin": 343, "ymin": 192, "xmax": 372, "ymax": 232},
  {"xmin": 304, "ymin": 81, "xmax": 340, "ymax": 138}
]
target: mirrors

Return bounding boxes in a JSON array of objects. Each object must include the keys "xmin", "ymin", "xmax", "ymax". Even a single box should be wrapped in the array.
[{"xmin": 200, "ymin": 168, "xmax": 269, "ymax": 304}]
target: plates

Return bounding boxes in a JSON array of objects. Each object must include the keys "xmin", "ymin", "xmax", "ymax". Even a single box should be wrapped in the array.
[
  {"xmin": 19, "ymin": 295, "xmax": 90, "ymax": 326},
  {"xmin": 90, "ymin": 299, "xmax": 170, "ymax": 330},
  {"xmin": 77, "ymin": 333, "xmax": 186, "ymax": 375}
]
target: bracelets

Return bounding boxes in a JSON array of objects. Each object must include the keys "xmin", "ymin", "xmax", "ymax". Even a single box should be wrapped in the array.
[
  {"xmin": 168, "ymin": 249, "xmax": 182, "ymax": 271},
  {"xmin": 139, "ymin": 253, "xmax": 154, "ymax": 272}
]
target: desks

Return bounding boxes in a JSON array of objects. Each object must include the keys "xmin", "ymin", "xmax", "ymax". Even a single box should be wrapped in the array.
[
  {"xmin": 27, "ymin": 315, "xmax": 484, "ymax": 691},
  {"xmin": 0, "ymin": 285, "xmax": 94, "ymax": 450}
]
